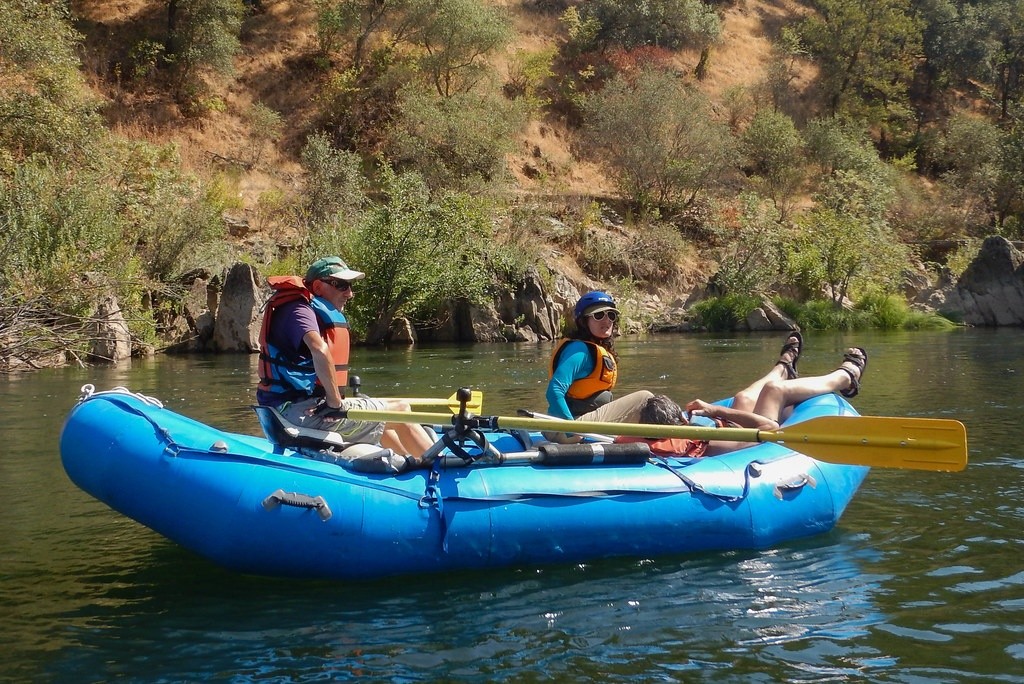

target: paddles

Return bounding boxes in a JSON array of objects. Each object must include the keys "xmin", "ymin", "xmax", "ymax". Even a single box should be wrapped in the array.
[
  {"xmin": 337, "ymin": 389, "xmax": 483, "ymax": 419},
  {"xmin": 318, "ymin": 406, "xmax": 969, "ymax": 474}
]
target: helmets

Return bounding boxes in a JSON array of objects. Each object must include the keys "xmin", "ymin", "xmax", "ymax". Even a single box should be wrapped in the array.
[{"xmin": 575, "ymin": 292, "xmax": 617, "ymax": 317}]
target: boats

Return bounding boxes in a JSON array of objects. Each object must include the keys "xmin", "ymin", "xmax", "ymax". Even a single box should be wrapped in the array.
[{"xmin": 58, "ymin": 382, "xmax": 872, "ymax": 581}]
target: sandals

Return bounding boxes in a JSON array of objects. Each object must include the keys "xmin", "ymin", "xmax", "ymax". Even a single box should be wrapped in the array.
[
  {"xmin": 837, "ymin": 347, "xmax": 866, "ymax": 398},
  {"xmin": 774, "ymin": 331, "xmax": 803, "ymax": 379}
]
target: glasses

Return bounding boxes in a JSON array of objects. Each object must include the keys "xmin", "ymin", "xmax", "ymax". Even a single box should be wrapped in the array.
[
  {"xmin": 319, "ymin": 278, "xmax": 353, "ymax": 291},
  {"xmin": 588, "ymin": 311, "xmax": 617, "ymax": 322}
]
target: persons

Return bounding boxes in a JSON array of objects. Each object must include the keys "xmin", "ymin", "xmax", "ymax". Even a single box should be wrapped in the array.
[
  {"xmin": 256, "ymin": 257, "xmax": 442, "ymax": 457},
  {"xmin": 541, "ymin": 291, "xmax": 655, "ymax": 443},
  {"xmin": 615, "ymin": 332, "xmax": 867, "ymax": 458}
]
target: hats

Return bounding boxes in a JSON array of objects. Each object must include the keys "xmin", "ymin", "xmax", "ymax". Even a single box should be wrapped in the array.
[
  {"xmin": 583, "ymin": 304, "xmax": 621, "ymax": 316},
  {"xmin": 304, "ymin": 256, "xmax": 365, "ymax": 286}
]
